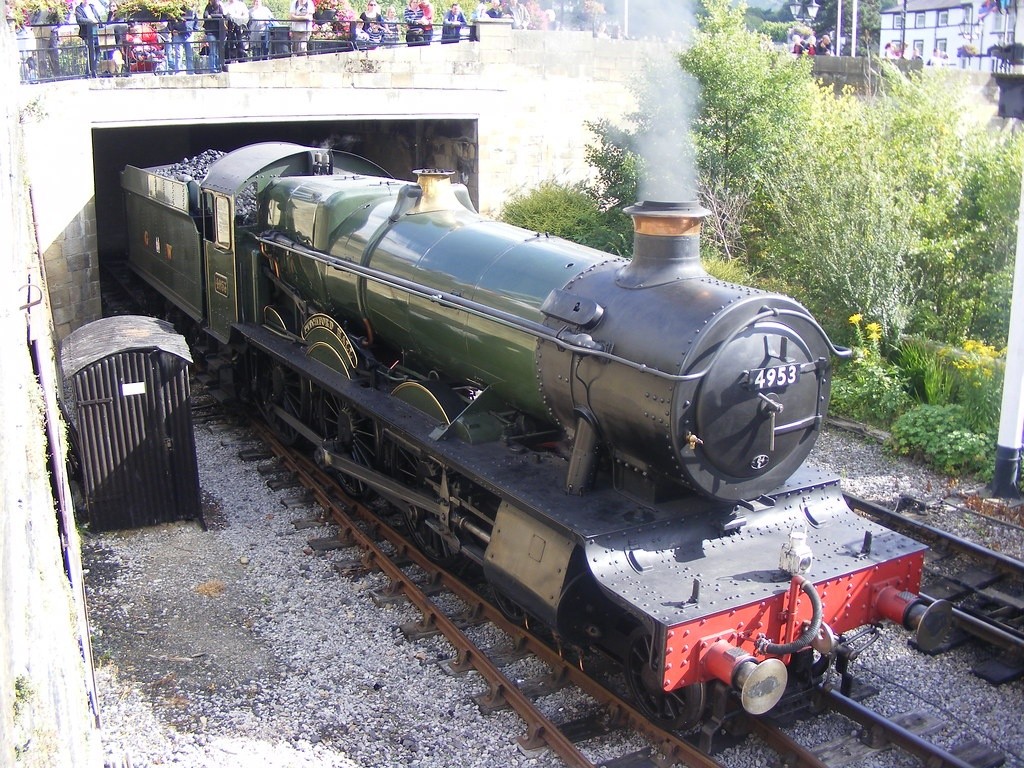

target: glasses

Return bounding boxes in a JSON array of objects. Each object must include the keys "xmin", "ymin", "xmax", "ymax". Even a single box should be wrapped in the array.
[
  {"xmin": 369, "ymin": 3, "xmax": 377, "ymax": 7},
  {"xmin": 413, "ymin": 2, "xmax": 418, "ymax": 4}
]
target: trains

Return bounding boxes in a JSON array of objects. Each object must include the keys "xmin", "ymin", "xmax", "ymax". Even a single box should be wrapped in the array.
[{"xmin": 122, "ymin": 140, "xmax": 952, "ymax": 755}]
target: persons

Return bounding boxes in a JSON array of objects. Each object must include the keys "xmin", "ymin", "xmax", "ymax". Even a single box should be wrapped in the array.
[
  {"xmin": 404, "ymin": 0, "xmax": 435, "ymax": 47},
  {"xmin": 441, "ymin": 2, "xmax": 467, "ymax": 45},
  {"xmin": 0, "ymin": 0, "xmax": 402, "ymax": 86},
  {"xmin": 469, "ymin": 0, "xmax": 635, "ymax": 43},
  {"xmin": 786, "ymin": 29, "xmax": 950, "ymax": 67}
]
